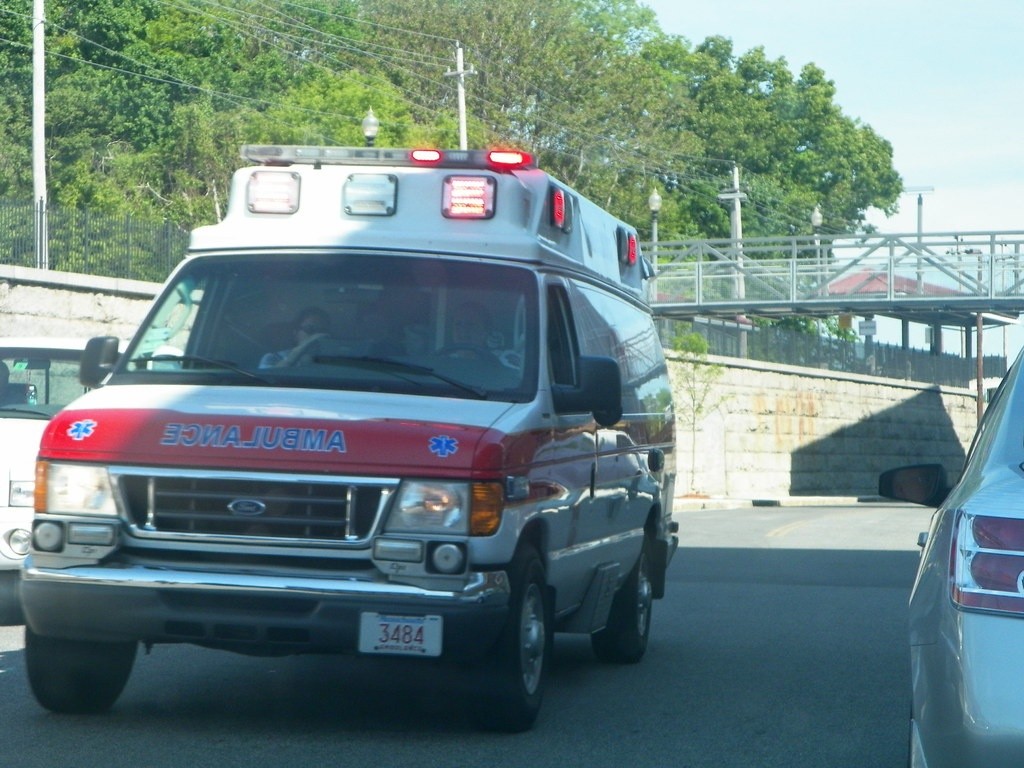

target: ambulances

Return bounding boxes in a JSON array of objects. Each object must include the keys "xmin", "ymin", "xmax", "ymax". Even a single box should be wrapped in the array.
[{"xmin": 18, "ymin": 147, "xmax": 680, "ymax": 734}]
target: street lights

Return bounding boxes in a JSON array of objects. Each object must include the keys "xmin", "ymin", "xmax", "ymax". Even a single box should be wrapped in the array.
[
  {"xmin": 809, "ymin": 206, "xmax": 822, "ymax": 299},
  {"xmin": 648, "ymin": 188, "xmax": 663, "ymax": 304}
]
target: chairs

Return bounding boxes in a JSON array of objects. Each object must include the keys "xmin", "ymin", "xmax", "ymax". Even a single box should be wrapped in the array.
[{"xmin": 240, "ymin": 324, "xmax": 333, "ymax": 372}]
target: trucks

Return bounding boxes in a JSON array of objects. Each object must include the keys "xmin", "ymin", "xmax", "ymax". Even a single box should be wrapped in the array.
[{"xmin": 0, "ymin": 332, "xmax": 183, "ymax": 625}]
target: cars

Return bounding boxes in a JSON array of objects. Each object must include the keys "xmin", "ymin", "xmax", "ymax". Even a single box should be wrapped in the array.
[{"xmin": 904, "ymin": 346, "xmax": 1023, "ymax": 768}]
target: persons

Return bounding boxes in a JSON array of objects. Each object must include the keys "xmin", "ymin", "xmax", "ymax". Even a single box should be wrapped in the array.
[
  {"xmin": 260, "ymin": 307, "xmax": 345, "ymax": 368},
  {"xmin": 451, "ymin": 301, "xmax": 515, "ymax": 368},
  {"xmin": 892, "ymin": 468, "xmax": 930, "ymax": 501}
]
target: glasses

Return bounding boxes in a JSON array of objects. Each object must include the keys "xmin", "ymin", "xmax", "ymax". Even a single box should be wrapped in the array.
[{"xmin": 299, "ymin": 325, "xmax": 328, "ymax": 335}]
